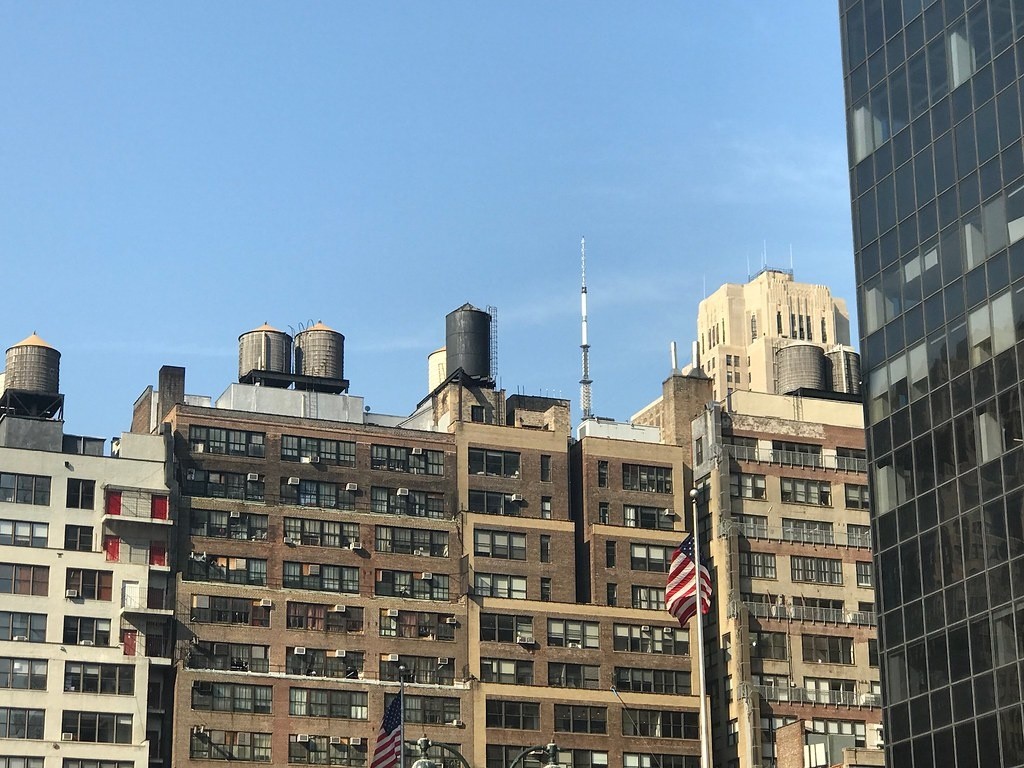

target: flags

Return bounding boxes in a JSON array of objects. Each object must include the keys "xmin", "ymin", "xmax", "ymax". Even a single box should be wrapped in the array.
[
  {"xmin": 369, "ymin": 689, "xmax": 402, "ymax": 768},
  {"xmin": 665, "ymin": 525, "xmax": 712, "ymax": 629}
]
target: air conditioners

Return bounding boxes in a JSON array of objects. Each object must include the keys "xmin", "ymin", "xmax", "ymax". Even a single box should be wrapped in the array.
[{"xmin": 13, "ymin": 442, "xmax": 676, "ymax": 746}]
